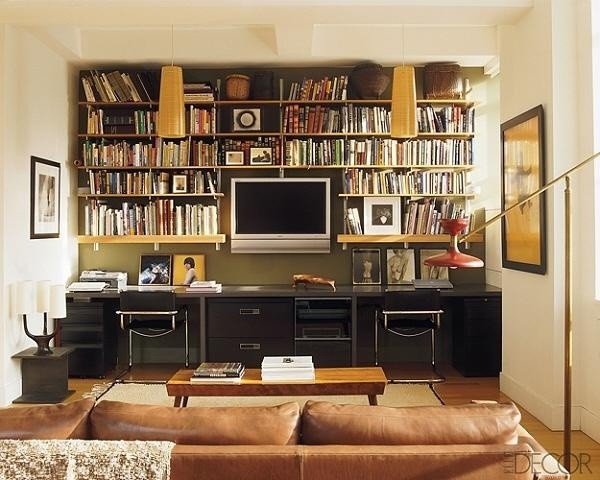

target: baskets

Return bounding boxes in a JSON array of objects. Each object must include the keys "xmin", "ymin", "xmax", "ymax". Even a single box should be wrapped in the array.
[{"xmin": 226, "ymin": 73, "xmax": 251, "ymax": 100}]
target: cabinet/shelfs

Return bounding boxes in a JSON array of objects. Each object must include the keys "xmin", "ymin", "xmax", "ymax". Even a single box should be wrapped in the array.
[
  {"xmin": 75, "ymin": 64, "xmax": 488, "ymax": 252},
  {"xmin": 205, "ymin": 296, "xmax": 296, "ymax": 370}
]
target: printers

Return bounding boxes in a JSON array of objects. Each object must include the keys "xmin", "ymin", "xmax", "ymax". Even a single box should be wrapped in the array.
[{"xmin": 67, "ymin": 270, "xmax": 128, "ymax": 294}]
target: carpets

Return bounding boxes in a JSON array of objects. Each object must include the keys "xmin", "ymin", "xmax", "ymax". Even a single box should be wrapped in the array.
[{"xmin": 83, "ymin": 377, "xmax": 447, "ymax": 409}]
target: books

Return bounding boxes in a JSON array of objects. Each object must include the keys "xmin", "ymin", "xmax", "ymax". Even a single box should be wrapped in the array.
[
  {"xmin": 189, "ymin": 362, "xmax": 246, "ymax": 383},
  {"xmin": 261, "ymin": 355, "xmax": 316, "ymax": 381},
  {"xmin": 186, "ymin": 278, "xmax": 223, "ymax": 293},
  {"xmin": 412, "ymin": 279, "xmax": 453, "ymax": 289}
]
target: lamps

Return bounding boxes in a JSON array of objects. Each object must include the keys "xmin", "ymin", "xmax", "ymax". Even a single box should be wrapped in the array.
[
  {"xmin": 14, "ymin": 278, "xmax": 68, "ymax": 356},
  {"xmin": 390, "ymin": 23, "xmax": 420, "ymax": 140},
  {"xmin": 156, "ymin": 24, "xmax": 187, "ymax": 139},
  {"xmin": 421, "ymin": 151, "xmax": 600, "ymax": 480}
]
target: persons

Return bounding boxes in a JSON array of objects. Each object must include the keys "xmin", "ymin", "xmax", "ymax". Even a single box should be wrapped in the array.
[
  {"xmin": 184, "ymin": 257, "xmax": 197, "ymax": 285},
  {"xmin": 361, "ymin": 257, "xmax": 372, "ymax": 283},
  {"xmin": 389, "ymin": 249, "xmax": 409, "ymax": 282}
]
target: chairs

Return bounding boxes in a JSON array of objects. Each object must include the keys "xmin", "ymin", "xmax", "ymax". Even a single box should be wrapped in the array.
[
  {"xmin": 369, "ymin": 288, "xmax": 449, "ymax": 384},
  {"xmin": 113, "ymin": 287, "xmax": 190, "ymax": 385}
]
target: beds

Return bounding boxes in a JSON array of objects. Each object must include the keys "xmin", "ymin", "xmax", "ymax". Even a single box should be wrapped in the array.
[{"xmin": 165, "ymin": 367, "xmax": 390, "ymax": 407}]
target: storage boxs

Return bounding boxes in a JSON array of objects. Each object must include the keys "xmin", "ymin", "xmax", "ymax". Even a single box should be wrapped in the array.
[
  {"xmin": 229, "ymin": 106, "xmax": 263, "ymax": 132},
  {"xmin": 102, "ymin": 115, "xmax": 136, "ymax": 135}
]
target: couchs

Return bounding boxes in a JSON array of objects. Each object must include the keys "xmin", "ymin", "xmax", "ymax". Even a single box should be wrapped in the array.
[{"xmin": 0, "ymin": 397, "xmax": 571, "ymax": 479}]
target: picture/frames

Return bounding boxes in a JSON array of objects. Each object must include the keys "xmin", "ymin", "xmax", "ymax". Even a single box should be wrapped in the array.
[
  {"xmin": 351, "ymin": 248, "xmax": 383, "ymax": 286},
  {"xmin": 225, "ymin": 151, "xmax": 245, "ymax": 166},
  {"xmin": 172, "ymin": 173, "xmax": 188, "ymax": 194},
  {"xmin": 137, "ymin": 254, "xmax": 173, "ymax": 287},
  {"xmin": 361, "ymin": 196, "xmax": 402, "ymax": 237},
  {"xmin": 499, "ymin": 102, "xmax": 547, "ymax": 275},
  {"xmin": 249, "ymin": 147, "xmax": 273, "ymax": 166},
  {"xmin": 28, "ymin": 154, "xmax": 62, "ymax": 240}
]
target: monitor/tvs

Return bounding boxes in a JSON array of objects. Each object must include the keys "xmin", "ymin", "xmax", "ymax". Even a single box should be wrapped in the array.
[{"xmin": 230, "ymin": 177, "xmax": 331, "ymax": 254}]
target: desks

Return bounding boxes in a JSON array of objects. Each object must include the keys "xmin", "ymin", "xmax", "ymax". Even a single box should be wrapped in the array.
[
  {"xmin": 62, "ymin": 285, "xmax": 503, "ymax": 378},
  {"xmin": 12, "ymin": 345, "xmax": 78, "ymax": 406}
]
